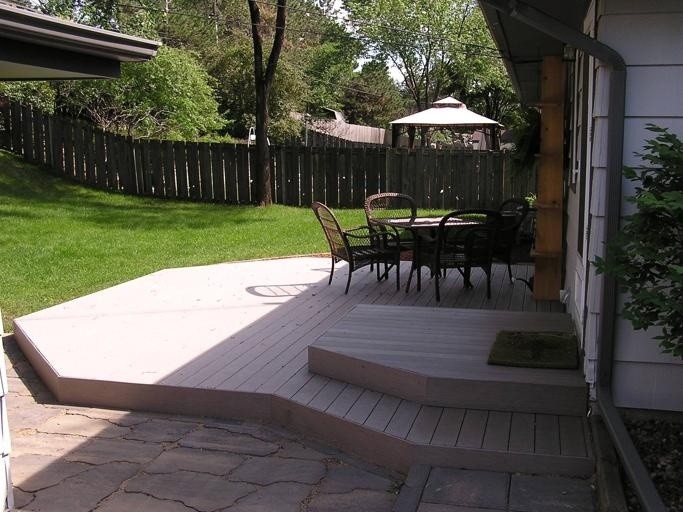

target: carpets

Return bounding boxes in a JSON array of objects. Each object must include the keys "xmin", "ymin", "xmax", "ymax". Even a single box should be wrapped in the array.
[{"xmin": 486, "ymin": 329, "xmax": 579, "ymax": 369}]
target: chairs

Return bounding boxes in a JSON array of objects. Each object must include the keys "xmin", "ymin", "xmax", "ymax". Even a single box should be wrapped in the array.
[
  {"xmin": 363, "ymin": 192, "xmax": 418, "ymax": 290},
  {"xmin": 405, "ymin": 208, "xmax": 502, "ymax": 304},
  {"xmin": 308, "ymin": 200, "xmax": 402, "ymax": 295},
  {"xmin": 464, "ymin": 195, "xmax": 528, "ymax": 289}
]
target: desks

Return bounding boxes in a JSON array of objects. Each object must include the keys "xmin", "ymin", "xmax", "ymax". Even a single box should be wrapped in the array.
[{"xmin": 370, "ymin": 213, "xmax": 487, "ymax": 294}]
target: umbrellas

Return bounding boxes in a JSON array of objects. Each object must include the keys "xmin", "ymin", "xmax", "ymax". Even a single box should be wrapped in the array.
[{"xmin": 383, "ymin": 94, "xmax": 503, "ymax": 150}]
